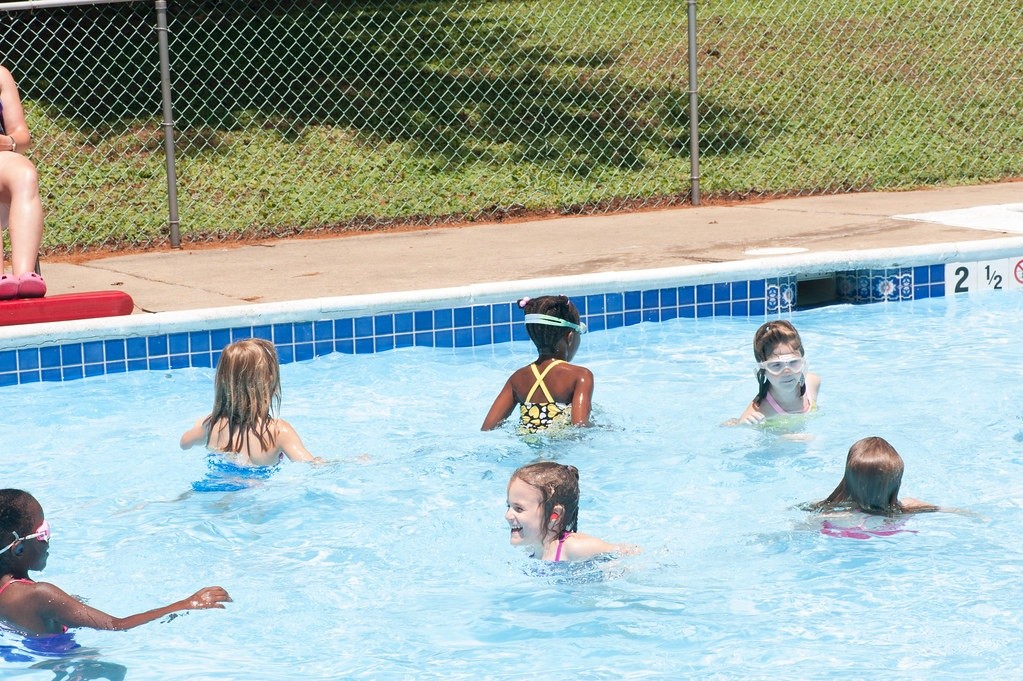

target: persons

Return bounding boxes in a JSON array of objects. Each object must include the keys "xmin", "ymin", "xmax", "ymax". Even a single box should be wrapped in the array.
[
  {"xmin": 820, "ymin": 436, "xmax": 924, "ymax": 511},
  {"xmin": 738, "ymin": 320, "xmax": 820, "ymax": 423},
  {"xmin": 505, "ymin": 462, "xmax": 623, "ymax": 561},
  {"xmin": 0, "ymin": 65, "xmax": 47, "ymax": 300},
  {"xmin": 0, "ymin": 489, "xmax": 233, "ymax": 635},
  {"xmin": 481, "ymin": 296, "xmax": 594, "ymax": 431},
  {"xmin": 180, "ymin": 338, "xmax": 315, "ymax": 464}
]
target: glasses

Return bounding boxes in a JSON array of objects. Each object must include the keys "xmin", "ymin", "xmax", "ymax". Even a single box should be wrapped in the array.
[
  {"xmin": 15, "ymin": 521, "xmax": 50, "ymax": 543},
  {"xmin": 566, "ymin": 322, "xmax": 587, "ymax": 334},
  {"xmin": 757, "ymin": 357, "xmax": 805, "ymax": 375}
]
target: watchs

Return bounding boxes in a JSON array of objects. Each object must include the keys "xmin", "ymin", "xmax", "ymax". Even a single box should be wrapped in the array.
[{"xmin": 10, "ymin": 136, "xmax": 17, "ymax": 152}]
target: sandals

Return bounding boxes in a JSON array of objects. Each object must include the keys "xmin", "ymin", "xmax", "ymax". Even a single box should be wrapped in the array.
[
  {"xmin": 0, "ymin": 274, "xmax": 19, "ymax": 299},
  {"xmin": 18, "ymin": 271, "xmax": 46, "ymax": 298}
]
